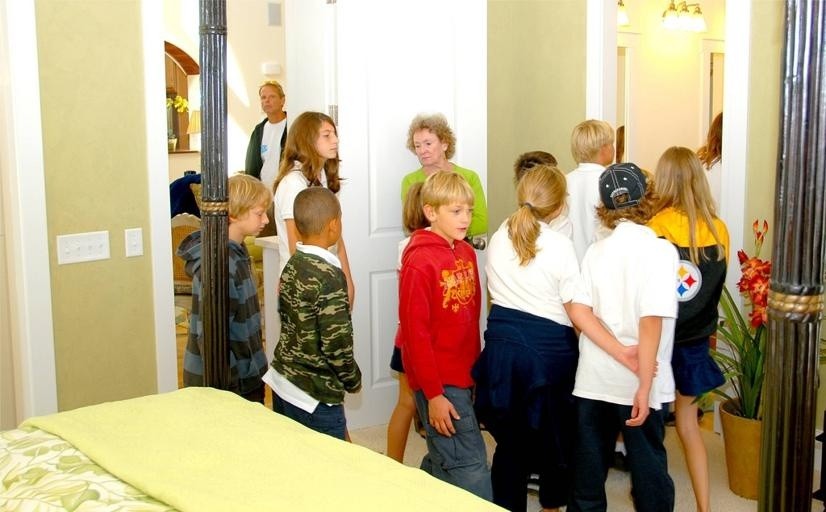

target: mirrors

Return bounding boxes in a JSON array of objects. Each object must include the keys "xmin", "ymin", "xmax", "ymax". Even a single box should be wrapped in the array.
[{"xmin": 616, "ymin": 43, "xmax": 629, "ymax": 166}]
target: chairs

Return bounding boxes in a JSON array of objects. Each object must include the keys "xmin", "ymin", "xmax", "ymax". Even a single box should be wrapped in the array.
[{"xmin": 170, "ymin": 213, "xmax": 203, "ymax": 294}]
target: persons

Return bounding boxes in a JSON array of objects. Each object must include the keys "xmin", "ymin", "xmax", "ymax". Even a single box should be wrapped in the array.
[
  {"xmin": 241, "ymin": 81, "xmax": 288, "ymax": 241},
  {"xmin": 272, "ymin": 111, "xmax": 356, "ymax": 313},
  {"xmin": 390, "ymin": 109, "xmax": 727, "ymax": 511},
  {"xmin": 174, "ymin": 174, "xmax": 268, "ymax": 405},
  {"xmin": 261, "ymin": 187, "xmax": 364, "ymax": 442}
]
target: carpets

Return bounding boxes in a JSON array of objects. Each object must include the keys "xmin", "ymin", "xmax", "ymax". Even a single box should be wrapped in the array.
[{"xmin": 348, "ymin": 405, "xmax": 826, "ymax": 512}]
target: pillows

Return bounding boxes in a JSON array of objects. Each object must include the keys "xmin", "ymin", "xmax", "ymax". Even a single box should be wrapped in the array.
[{"xmin": 190, "ymin": 182, "xmax": 203, "ymax": 214}]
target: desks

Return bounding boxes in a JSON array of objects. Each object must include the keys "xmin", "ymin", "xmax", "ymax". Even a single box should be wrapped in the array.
[{"xmin": 254, "ymin": 235, "xmax": 282, "ymax": 367}]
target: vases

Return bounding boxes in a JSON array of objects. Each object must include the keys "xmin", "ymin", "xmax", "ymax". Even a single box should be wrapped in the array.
[
  {"xmin": 717, "ymin": 397, "xmax": 763, "ymax": 499},
  {"xmin": 167, "ymin": 138, "xmax": 177, "ymax": 152}
]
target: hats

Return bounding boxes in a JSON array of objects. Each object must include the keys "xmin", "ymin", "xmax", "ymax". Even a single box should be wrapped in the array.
[{"xmin": 598, "ymin": 161, "xmax": 653, "ymax": 209}]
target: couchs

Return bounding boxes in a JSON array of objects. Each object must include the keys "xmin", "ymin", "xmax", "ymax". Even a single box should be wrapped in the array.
[{"xmin": 169, "ymin": 173, "xmax": 205, "ymax": 218}]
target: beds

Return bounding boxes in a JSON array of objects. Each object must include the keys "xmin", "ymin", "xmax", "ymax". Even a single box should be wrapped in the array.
[{"xmin": 0, "ymin": 386, "xmax": 511, "ymax": 512}]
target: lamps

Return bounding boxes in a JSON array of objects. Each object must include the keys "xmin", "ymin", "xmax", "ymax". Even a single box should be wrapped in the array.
[
  {"xmin": 616, "ymin": 0, "xmax": 629, "ymax": 25},
  {"xmin": 657, "ymin": 0, "xmax": 707, "ymax": 34},
  {"xmin": 186, "ymin": 110, "xmax": 203, "ymax": 134}
]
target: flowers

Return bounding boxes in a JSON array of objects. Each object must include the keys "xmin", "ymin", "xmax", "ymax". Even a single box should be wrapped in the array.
[
  {"xmin": 698, "ymin": 219, "xmax": 774, "ymax": 418},
  {"xmin": 166, "ymin": 96, "xmax": 190, "ymax": 113}
]
